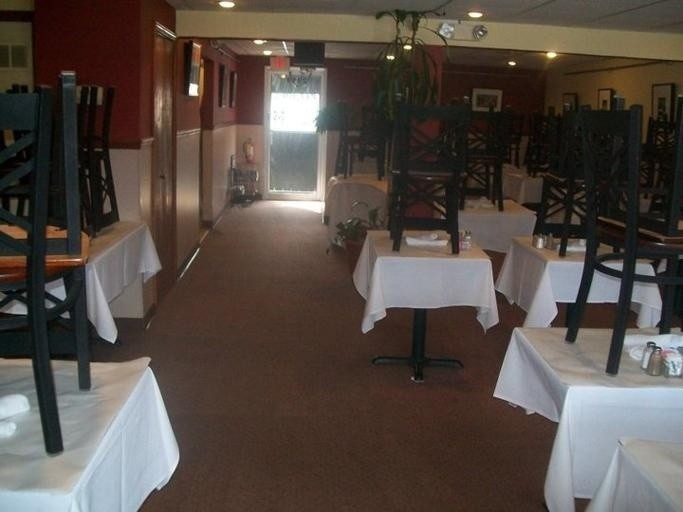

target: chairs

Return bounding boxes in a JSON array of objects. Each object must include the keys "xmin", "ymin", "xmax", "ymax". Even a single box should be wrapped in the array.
[
  {"xmin": 567, "ymin": 103, "xmax": 683, "ymax": 375},
  {"xmin": 3, "ymin": 70, "xmax": 122, "ymax": 457},
  {"xmin": 335, "ymin": 100, "xmax": 682, "ymax": 264}
]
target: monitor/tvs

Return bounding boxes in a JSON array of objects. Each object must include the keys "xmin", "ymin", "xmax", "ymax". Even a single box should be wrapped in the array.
[{"xmin": 293, "ymin": 41, "xmax": 325, "ymax": 69}]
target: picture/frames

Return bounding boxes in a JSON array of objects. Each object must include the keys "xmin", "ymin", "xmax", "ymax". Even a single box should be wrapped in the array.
[
  {"xmin": 651, "ymin": 83, "xmax": 675, "ymax": 121},
  {"xmin": 598, "ymin": 89, "xmax": 613, "ymax": 111},
  {"xmin": 470, "ymin": 86, "xmax": 504, "ymax": 114},
  {"xmin": 562, "ymin": 93, "xmax": 578, "ymax": 112}
]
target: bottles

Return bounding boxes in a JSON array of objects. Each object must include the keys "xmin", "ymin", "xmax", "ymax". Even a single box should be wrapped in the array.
[
  {"xmin": 643, "ymin": 340, "xmax": 662, "ymax": 377},
  {"xmin": 536, "ymin": 233, "xmax": 554, "ymax": 249}
]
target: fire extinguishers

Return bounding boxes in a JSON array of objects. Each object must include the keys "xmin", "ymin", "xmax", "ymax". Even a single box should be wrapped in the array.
[{"xmin": 243, "ymin": 138, "xmax": 255, "ymax": 164}]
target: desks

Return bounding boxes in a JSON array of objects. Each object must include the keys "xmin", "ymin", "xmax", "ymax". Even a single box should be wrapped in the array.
[
  {"xmin": 1, "ymin": 221, "xmax": 161, "ymax": 350},
  {"xmin": 492, "ymin": 233, "xmax": 670, "ymax": 331},
  {"xmin": 326, "ymin": 174, "xmax": 391, "ymax": 249},
  {"xmin": 2, "ymin": 355, "xmax": 181, "ymax": 512},
  {"xmin": 492, "ymin": 327, "xmax": 683, "ymax": 511},
  {"xmin": 351, "ymin": 228, "xmax": 502, "ymax": 383}
]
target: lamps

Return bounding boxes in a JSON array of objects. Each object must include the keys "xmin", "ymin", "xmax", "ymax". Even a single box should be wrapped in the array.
[{"xmin": 439, "ymin": 22, "xmax": 489, "ymax": 41}]
